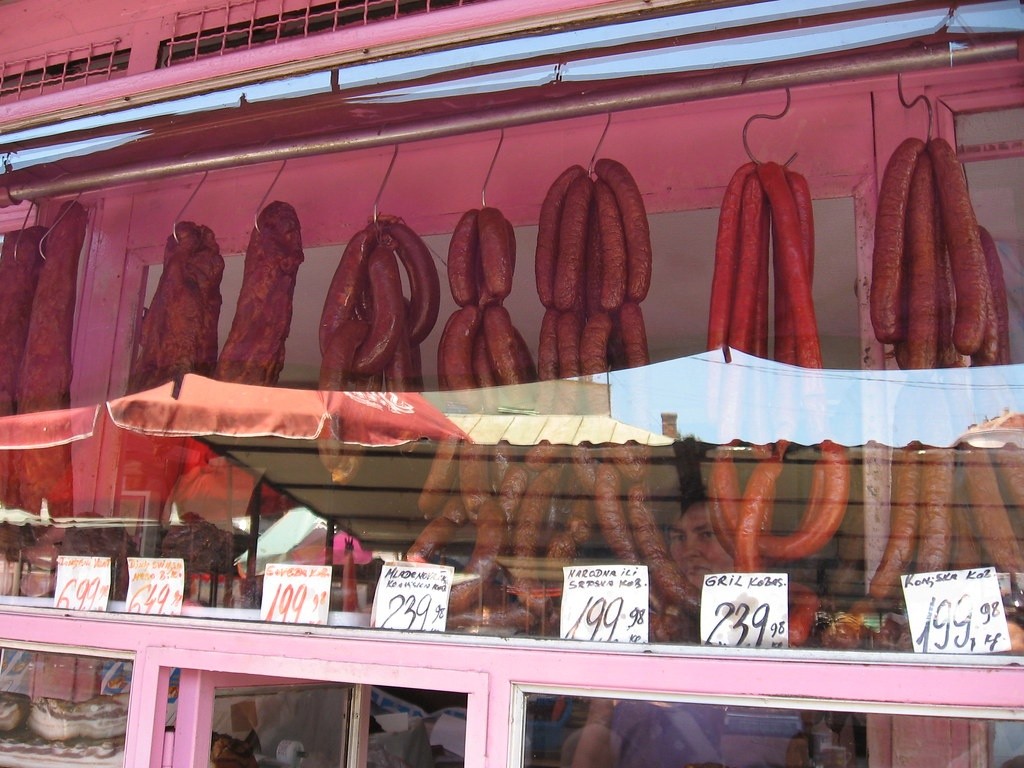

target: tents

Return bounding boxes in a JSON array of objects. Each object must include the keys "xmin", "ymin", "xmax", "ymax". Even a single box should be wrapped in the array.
[{"xmin": 188, "ymin": 412, "xmax": 1024, "ymax": 609}]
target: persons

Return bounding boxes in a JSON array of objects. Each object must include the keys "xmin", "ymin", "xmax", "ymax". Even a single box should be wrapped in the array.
[{"xmin": 573, "ymin": 483, "xmax": 810, "ymax": 768}]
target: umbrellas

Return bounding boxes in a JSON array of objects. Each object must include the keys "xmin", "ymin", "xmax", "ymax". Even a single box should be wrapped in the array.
[{"xmin": 0, "ymin": 373, "xmax": 475, "ymax": 579}]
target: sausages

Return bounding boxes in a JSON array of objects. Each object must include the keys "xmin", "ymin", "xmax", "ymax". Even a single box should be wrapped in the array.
[{"xmin": 316, "ymin": 138, "xmax": 1024, "ymax": 647}]
